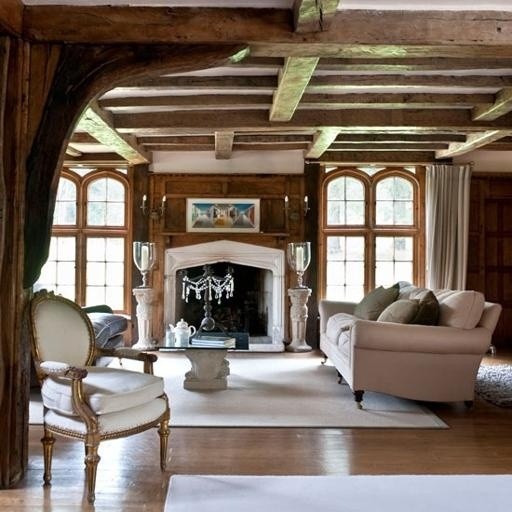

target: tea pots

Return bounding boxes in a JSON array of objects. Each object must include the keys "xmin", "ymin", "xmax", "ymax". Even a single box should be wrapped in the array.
[{"xmin": 168, "ymin": 318, "xmax": 196, "ymax": 347}]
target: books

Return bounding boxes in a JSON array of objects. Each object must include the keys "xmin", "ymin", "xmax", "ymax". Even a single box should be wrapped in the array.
[
  {"xmin": 192, "ymin": 335, "xmax": 236, "ymax": 343},
  {"xmin": 192, "ymin": 341, "xmax": 235, "ymax": 346}
]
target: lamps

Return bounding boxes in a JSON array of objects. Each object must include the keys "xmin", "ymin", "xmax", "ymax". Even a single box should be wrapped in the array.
[
  {"xmin": 284, "ymin": 196, "xmax": 308, "ymax": 221},
  {"xmin": 139, "ymin": 193, "xmax": 167, "ymax": 220},
  {"xmin": 180, "ymin": 265, "xmax": 236, "ymax": 339}
]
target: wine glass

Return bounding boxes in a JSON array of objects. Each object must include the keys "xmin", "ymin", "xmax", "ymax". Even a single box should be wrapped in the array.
[
  {"xmin": 286, "ymin": 240, "xmax": 312, "ymax": 290},
  {"xmin": 131, "ymin": 240, "xmax": 157, "ymax": 290}
]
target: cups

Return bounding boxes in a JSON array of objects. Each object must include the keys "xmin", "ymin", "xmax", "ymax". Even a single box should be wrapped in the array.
[{"xmin": 165, "ymin": 328, "xmax": 176, "ymax": 348}]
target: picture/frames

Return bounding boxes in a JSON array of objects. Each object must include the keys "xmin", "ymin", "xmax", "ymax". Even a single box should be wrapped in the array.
[{"xmin": 185, "ymin": 197, "xmax": 261, "ymax": 233}]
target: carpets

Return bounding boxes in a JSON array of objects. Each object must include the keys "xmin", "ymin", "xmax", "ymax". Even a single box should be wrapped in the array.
[
  {"xmin": 474, "ymin": 365, "xmax": 512, "ymax": 407},
  {"xmin": 29, "ymin": 349, "xmax": 450, "ymax": 428},
  {"xmin": 163, "ymin": 475, "xmax": 512, "ymax": 512}
]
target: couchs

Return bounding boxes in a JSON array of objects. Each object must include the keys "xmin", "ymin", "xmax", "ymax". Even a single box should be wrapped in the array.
[{"xmin": 317, "ymin": 302, "xmax": 503, "ymax": 409}]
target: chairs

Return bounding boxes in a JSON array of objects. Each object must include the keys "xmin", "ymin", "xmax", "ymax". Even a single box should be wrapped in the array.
[
  {"xmin": 31, "ymin": 304, "xmax": 128, "ymax": 392},
  {"xmin": 25, "ymin": 289, "xmax": 171, "ymax": 504}
]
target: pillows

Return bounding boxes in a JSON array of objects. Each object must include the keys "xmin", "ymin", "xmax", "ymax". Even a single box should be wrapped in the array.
[
  {"xmin": 86, "ymin": 310, "xmax": 127, "ymax": 348},
  {"xmin": 42, "ymin": 367, "xmax": 164, "ymax": 416},
  {"xmin": 354, "ymin": 281, "xmax": 486, "ymax": 329}
]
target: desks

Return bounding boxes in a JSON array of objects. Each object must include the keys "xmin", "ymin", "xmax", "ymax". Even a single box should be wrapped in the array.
[{"xmin": 157, "ymin": 332, "xmax": 249, "ymax": 391}]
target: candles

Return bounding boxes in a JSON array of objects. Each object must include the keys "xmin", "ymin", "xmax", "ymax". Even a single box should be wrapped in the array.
[
  {"xmin": 296, "ymin": 247, "xmax": 305, "ymax": 273},
  {"xmin": 141, "ymin": 246, "xmax": 148, "ymax": 271}
]
